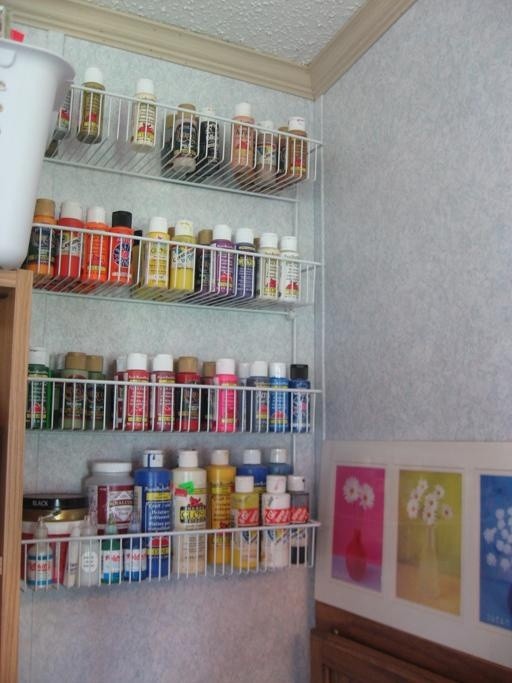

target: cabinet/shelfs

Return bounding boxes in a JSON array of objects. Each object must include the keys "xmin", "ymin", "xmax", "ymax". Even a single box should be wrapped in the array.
[{"xmin": 21, "ymin": 82, "xmax": 323, "ymax": 593}]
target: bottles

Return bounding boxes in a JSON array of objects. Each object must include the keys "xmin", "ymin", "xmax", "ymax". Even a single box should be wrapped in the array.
[
  {"xmin": 230, "ymin": 102, "xmax": 310, "ymax": 193},
  {"xmin": 77, "ymin": 66, "xmax": 106, "ymax": 144},
  {"xmin": 162, "ymin": 101, "xmax": 224, "ymax": 183},
  {"xmin": 24, "ymin": 198, "xmax": 302, "ymax": 309},
  {"xmin": 46, "ymin": 90, "xmax": 72, "ymax": 159},
  {"xmin": 25, "ymin": 348, "xmax": 315, "ymax": 433},
  {"xmin": 20, "ymin": 447, "xmax": 310, "ymax": 590},
  {"xmin": 131, "ymin": 77, "xmax": 158, "ymax": 154}
]
